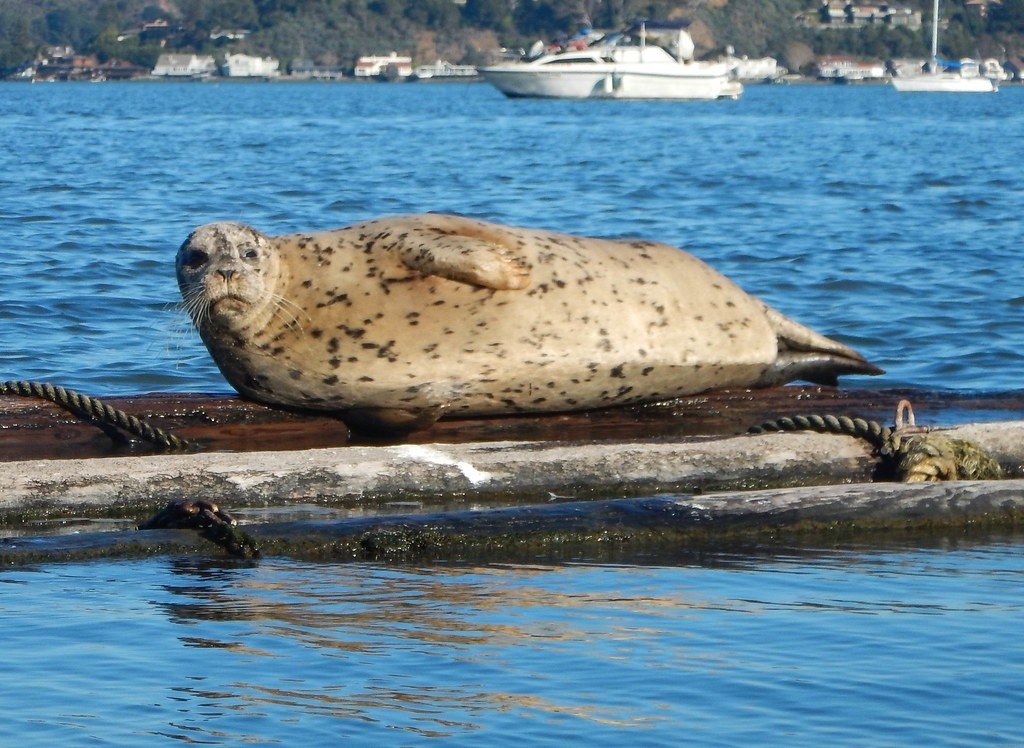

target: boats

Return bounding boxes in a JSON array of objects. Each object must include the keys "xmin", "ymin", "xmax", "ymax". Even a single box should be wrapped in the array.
[
  {"xmin": 474, "ymin": 18, "xmax": 745, "ymax": 100},
  {"xmin": 888, "ymin": 57, "xmax": 998, "ymax": 93}
]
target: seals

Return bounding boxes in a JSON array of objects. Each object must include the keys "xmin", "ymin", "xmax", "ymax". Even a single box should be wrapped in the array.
[{"xmin": 174, "ymin": 211, "xmax": 888, "ymax": 440}]
